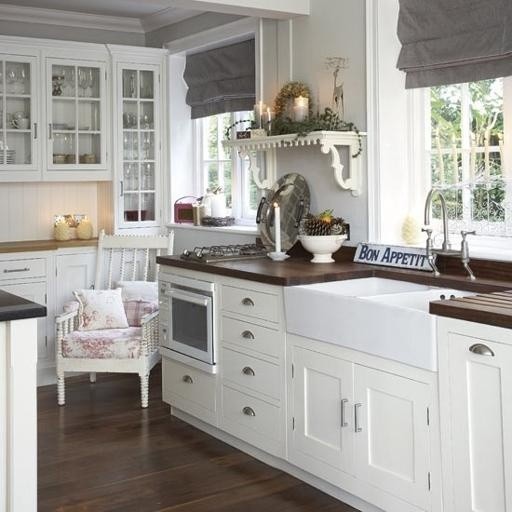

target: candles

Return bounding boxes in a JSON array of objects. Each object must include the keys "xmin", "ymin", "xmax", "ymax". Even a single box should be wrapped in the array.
[{"xmin": 254, "ymin": 106, "xmax": 309, "ymax": 132}]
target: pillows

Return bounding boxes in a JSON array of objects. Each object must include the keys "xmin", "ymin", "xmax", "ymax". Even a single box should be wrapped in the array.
[
  {"xmin": 73, "ymin": 287, "xmax": 130, "ymax": 332},
  {"xmin": 122, "ymin": 297, "xmax": 158, "ymax": 326}
]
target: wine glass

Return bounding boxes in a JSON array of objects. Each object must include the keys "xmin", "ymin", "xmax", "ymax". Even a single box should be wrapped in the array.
[
  {"xmin": 0, "ymin": 62, "xmax": 97, "ymax": 100},
  {"xmin": 297, "ymin": 234, "xmax": 347, "ymax": 264},
  {"xmin": 123, "ymin": 72, "xmax": 152, "ymax": 192}
]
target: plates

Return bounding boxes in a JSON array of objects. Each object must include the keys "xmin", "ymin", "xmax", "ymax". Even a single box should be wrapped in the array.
[
  {"xmin": 0, "ymin": 148, "xmax": 16, "ymax": 165},
  {"xmin": 53, "ymin": 124, "xmax": 70, "ymax": 130}
]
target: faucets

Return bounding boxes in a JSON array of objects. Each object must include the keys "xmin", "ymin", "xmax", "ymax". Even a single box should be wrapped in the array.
[{"xmin": 423, "ymin": 188, "xmax": 452, "ymax": 252}]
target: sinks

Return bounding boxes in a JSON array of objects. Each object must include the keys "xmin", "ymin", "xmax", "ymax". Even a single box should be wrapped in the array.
[
  {"xmin": 280, "ymin": 275, "xmax": 430, "ymax": 350},
  {"xmin": 358, "ymin": 289, "xmax": 486, "ymax": 372}
]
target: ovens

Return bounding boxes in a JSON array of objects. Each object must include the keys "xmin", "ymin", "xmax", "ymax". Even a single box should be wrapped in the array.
[{"xmin": 157, "ymin": 272, "xmax": 216, "ymax": 375}]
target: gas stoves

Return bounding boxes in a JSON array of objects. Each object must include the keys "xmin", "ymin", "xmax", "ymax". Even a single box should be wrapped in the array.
[{"xmin": 181, "ymin": 242, "xmax": 274, "ymax": 263}]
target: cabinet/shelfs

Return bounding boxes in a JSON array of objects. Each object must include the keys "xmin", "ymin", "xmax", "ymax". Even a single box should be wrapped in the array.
[
  {"xmin": 98, "ymin": 43, "xmax": 173, "ymax": 235},
  {"xmin": 436, "ymin": 316, "xmax": 512, "ymax": 512},
  {"xmin": 0, "ymin": 249, "xmax": 54, "ymax": 388},
  {"xmin": 287, "ymin": 332, "xmax": 444, "ymax": 512},
  {"xmin": 0, "ymin": 34, "xmax": 112, "ymax": 182},
  {"xmin": 54, "ymin": 247, "xmax": 155, "ymax": 385},
  {"xmin": 217, "ymin": 276, "xmax": 287, "ymax": 473}
]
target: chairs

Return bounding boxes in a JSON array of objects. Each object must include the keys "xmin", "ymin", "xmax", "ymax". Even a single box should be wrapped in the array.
[{"xmin": 54, "ymin": 228, "xmax": 176, "ymax": 409}]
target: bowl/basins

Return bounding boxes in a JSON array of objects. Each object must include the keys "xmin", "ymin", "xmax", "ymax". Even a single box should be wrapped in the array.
[
  {"xmin": 82, "ymin": 154, "xmax": 97, "ymax": 163},
  {"xmin": 126, "ymin": 210, "xmax": 146, "ymax": 221},
  {"xmin": 54, "ymin": 155, "xmax": 66, "ymax": 163},
  {"xmin": 68, "ymin": 155, "xmax": 81, "ymax": 163}
]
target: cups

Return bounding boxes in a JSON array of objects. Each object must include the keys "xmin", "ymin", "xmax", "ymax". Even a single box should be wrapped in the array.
[{"xmin": 0, "ymin": 110, "xmax": 29, "ymax": 130}]
